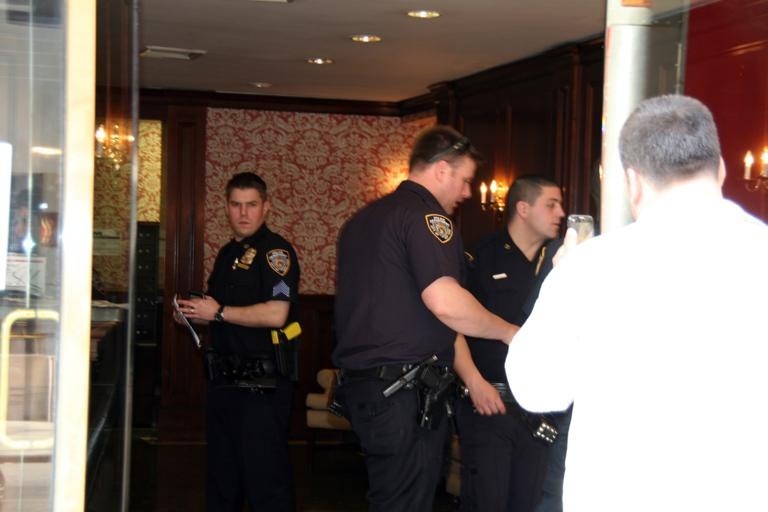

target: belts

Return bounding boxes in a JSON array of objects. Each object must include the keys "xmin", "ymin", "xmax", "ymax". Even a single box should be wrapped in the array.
[{"xmin": 341, "ymin": 363, "xmax": 399, "ymax": 382}]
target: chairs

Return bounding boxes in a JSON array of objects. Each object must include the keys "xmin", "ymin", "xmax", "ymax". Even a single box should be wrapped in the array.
[
  {"xmin": 443, "ymin": 434, "xmax": 461, "ymax": 512},
  {"xmin": 305, "ymin": 368, "xmax": 353, "ymax": 472}
]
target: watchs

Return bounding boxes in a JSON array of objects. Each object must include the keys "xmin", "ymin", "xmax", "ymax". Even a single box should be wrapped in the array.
[{"xmin": 214, "ymin": 304, "xmax": 224, "ymax": 324}]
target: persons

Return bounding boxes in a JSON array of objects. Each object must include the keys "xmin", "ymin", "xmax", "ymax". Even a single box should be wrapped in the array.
[
  {"xmin": 446, "ymin": 174, "xmax": 566, "ymax": 510},
  {"xmin": 332, "ymin": 123, "xmax": 519, "ymax": 510},
  {"xmin": 504, "ymin": 93, "xmax": 768, "ymax": 512},
  {"xmin": 171, "ymin": 171, "xmax": 305, "ymax": 511}
]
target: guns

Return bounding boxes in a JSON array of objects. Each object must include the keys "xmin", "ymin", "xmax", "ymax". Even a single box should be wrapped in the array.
[
  {"xmin": 417, "ymin": 365, "xmax": 459, "ymax": 431},
  {"xmin": 270, "ymin": 321, "xmax": 303, "ymax": 377}
]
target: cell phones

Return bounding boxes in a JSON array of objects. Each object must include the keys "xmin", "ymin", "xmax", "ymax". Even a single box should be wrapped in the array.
[
  {"xmin": 189, "ymin": 290, "xmax": 209, "ymax": 325},
  {"xmin": 567, "ymin": 214, "xmax": 595, "ymax": 245}
]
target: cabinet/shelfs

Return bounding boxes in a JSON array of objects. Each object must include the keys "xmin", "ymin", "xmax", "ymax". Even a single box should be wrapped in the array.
[{"xmin": 134, "ymin": 222, "xmax": 160, "ymax": 428}]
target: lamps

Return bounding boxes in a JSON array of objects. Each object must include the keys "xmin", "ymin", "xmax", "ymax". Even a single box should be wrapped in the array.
[
  {"xmin": 96, "ymin": 119, "xmax": 135, "ymax": 171},
  {"xmin": 479, "ymin": 179, "xmax": 510, "ymax": 217},
  {"xmin": 742, "ymin": 145, "xmax": 768, "ymax": 193}
]
